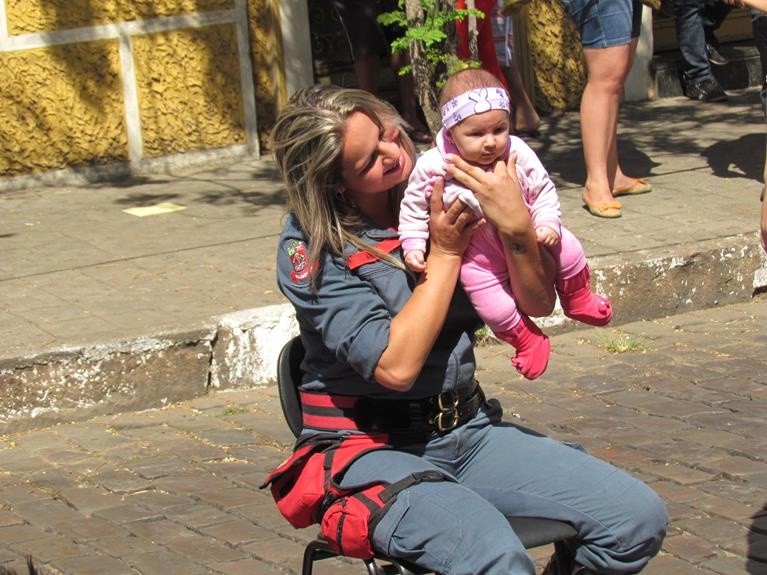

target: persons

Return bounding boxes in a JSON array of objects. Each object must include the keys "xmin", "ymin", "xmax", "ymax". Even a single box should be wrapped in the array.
[
  {"xmin": 672, "ymin": 1, "xmax": 740, "ymax": 101},
  {"xmin": 560, "ymin": 1, "xmax": 653, "ymax": 218},
  {"xmin": 356, "ymin": 0, "xmax": 433, "ymax": 142},
  {"xmin": 397, "ymin": 69, "xmax": 614, "ymax": 380},
  {"xmin": 272, "ymin": 84, "xmax": 668, "ymax": 575}
]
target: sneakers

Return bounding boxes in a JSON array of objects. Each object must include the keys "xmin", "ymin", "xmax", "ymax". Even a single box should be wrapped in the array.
[
  {"xmin": 685, "ymin": 78, "xmax": 728, "ymax": 102},
  {"xmin": 703, "ymin": 33, "xmax": 729, "ymax": 65}
]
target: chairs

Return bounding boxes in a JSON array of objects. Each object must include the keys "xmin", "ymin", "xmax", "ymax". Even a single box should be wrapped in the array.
[{"xmin": 275, "ymin": 333, "xmax": 579, "ymax": 575}]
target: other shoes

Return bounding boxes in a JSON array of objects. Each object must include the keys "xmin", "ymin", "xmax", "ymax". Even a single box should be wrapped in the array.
[
  {"xmin": 580, "ymin": 185, "xmax": 622, "ymax": 217},
  {"xmin": 509, "ymin": 319, "xmax": 551, "ymax": 381},
  {"xmin": 610, "ymin": 178, "xmax": 652, "ymax": 196},
  {"xmin": 559, "ymin": 282, "xmax": 613, "ymax": 327}
]
